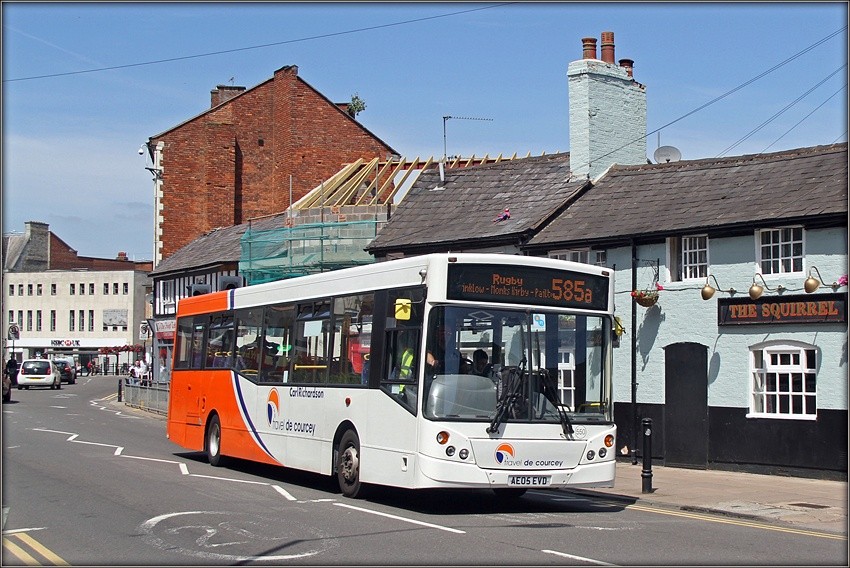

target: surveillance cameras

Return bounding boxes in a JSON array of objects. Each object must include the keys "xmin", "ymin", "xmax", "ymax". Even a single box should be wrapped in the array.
[{"xmin": 138, "ymin": 149, "xmax": 144, "ymax": 155}]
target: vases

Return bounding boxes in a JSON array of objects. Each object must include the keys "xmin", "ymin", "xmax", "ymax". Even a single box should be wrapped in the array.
[{"xmin": 631, "ymin": 296, "xmax": 658, "ymax": 308}]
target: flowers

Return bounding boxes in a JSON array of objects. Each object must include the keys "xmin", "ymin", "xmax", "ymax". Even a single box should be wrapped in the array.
[{"xmin": 627, "ymin": 282, "xmax": 664, "ymax": 298}]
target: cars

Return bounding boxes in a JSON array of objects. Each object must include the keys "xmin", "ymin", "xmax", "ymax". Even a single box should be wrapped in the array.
[
  {"xmin": 52, "ymin": 360, "xmax": 77, "ymax": 385},
  {"xmin": 16, "ymin": 359, "xmax": 62, "ymax": 390},
  {"xmin": 5, "ymin": 363, "xmax": 22, "ymax": 388}
]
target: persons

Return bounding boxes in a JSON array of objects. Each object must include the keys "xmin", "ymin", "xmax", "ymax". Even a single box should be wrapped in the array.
[
  {"xmin": 129, "ymin": 355, "xmax": 150, "ymax": 386},
  {"xmin": 3, "ymin": 355, "xmax": 17, "ymax": 386},
  {"xmin": 86, "ymin": 360, "xmax": 96, "ymax": 377},
  {"xmin": 393, "ymin": 317, "xmax": 491, "ymax": 409}
]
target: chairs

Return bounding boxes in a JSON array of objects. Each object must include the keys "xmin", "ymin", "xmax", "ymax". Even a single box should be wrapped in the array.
[{"xmin": 190, "ymin": 346, "xmax": 371, "ymax": 387}]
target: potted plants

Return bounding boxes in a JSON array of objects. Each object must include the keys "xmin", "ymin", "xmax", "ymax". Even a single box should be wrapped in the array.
[{"xmin": 559, "ymin": 315, "xmax": 576, "ymax": 330}]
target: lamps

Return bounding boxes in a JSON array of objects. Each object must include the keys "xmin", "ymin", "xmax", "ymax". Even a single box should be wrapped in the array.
[
  {"xmin": 749, "ymin": 272, "xmax": 785, "ymax": 301},
  {"xmin": 701, "ymin": 274, "xmax": 736, "ymax": 300},
  {"xmin": 805, "ymin": 266, "xmax": 840, "ymax": 293}
]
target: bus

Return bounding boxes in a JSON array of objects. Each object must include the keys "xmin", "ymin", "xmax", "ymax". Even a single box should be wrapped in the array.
[{"xmin": 163, "ymin": 251, "xmax": 627, "ymax": 499}]
